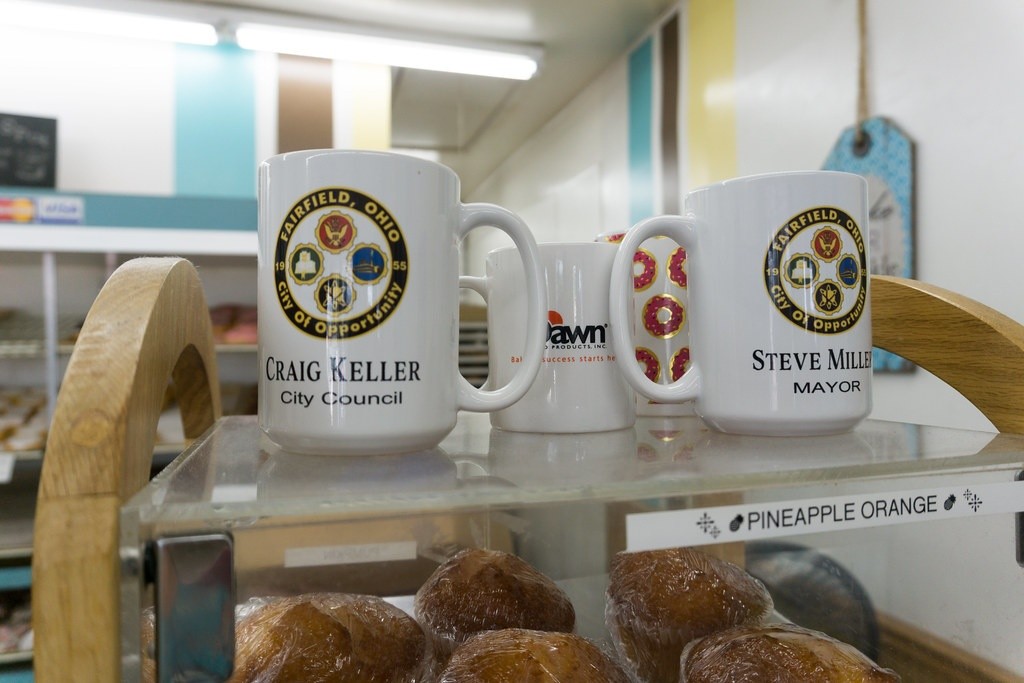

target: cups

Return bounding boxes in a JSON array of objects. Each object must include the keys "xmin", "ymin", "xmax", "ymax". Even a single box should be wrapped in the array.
[
  {"xmin": 458, "ymin": 242, "xmax": 634, "ymax": 435},
  {"xmin": 257, "ymin": 148, "xmax": 546, "ymax": 453},
  {"xmin": 608, "ymin": 171, "xmax": 873, "ymax": 436},
  {"xmin": 596, "ymin": 233, "xmax": 696, "ymax": 420}
]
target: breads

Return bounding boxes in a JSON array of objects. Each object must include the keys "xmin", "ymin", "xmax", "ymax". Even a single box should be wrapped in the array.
[
  {"xmin": 0, "ymin": 381, "xmax": 74, "ymax": 450},
  {"xmin": 0, "ymin": 586, "xmax": 36, "ymax": 655},
  {"xmin": 207, "ymin": 302, "xmax": 259, "ymax": 346},
  {"xmin": 138, "ymin": 545, "xmax": 907, "ymax": 683}
]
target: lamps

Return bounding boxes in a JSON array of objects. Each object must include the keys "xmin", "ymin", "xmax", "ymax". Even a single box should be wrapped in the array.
[{"xmin": 0, "ymin": 0, "xmax": 546, "ymax": 80}]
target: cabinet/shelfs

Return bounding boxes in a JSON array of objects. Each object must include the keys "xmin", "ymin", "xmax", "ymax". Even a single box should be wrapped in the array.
[{"xmin": 0, "ymin": 228, "xmax": 260, "ymax": 664}]
